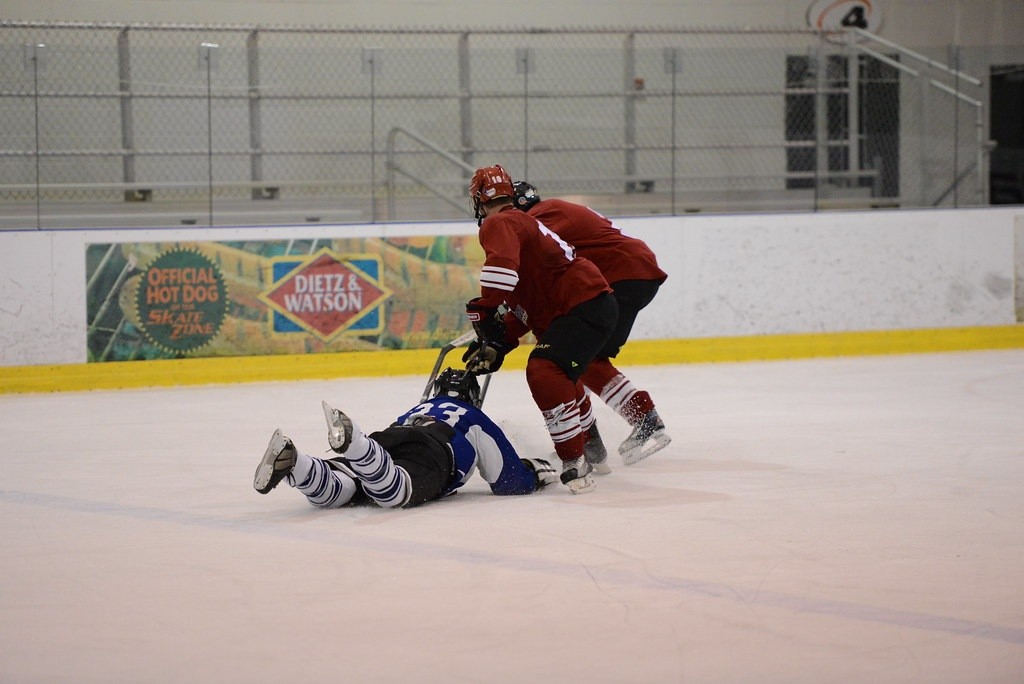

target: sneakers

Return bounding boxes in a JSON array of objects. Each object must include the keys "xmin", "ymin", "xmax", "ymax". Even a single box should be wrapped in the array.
[
  {"xmin": 254, "ymin": 428, "xmax": 298, "ymax": 494},
  {"xmin": 560, "ymin": 454, "xmax": 597, "ymax": 495},
  {"xmin": 619, "ymin": 408, "xmax": 672, "ymax": 465},
  {"xmin": 322, "ymin": 400, "xmax": 353, "ymax": 454},
  {"xmin": 584, "ymin": 418, "xmax": 613, "ymax": 474}
]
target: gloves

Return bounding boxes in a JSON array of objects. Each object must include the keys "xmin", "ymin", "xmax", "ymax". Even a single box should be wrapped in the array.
[
  {"xmin": 462, "ymin": 336, "xmax": 518, "ymax": 376},
  {"xmin": 519, "ymin": 458, "xmax": 559, "ymax": 491},
  {"xmin": 465, "ymin": 297, "xmax": 506, "ymax": 342}
]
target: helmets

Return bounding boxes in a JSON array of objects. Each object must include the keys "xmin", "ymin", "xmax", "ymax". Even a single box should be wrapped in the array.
[
  {"xmin": 433, "ymin": 367, "xmax": 481, "ymax": 407},
  {"xmin": 512, "ymin": 181, "xmax": 540, "ymax": 212},
  {"xmin": 469, "ymin": 165, "xmax": 516, "ymax": 205}
]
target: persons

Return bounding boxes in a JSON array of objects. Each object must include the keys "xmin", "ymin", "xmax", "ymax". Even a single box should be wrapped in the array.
[
  {"xmin": 460, "ymin": 164, "xmax": 618, "ymax": 493},
  {"xmin": 253, "ymin": 366, "xmax": 559, "ymax": 510},
  {"xmin": 512, "ymin": 180, "xmax": 670, "ymax": 475}
]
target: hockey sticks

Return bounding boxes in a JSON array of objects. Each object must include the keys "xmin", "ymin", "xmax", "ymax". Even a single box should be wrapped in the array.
[
  {"xmin": 420, "ymin": 329, "xmax": 479, "ymax": 403},
  {"xmin": 476, "ymin": 373, "xmax": 491, "ymax": 410}
]
